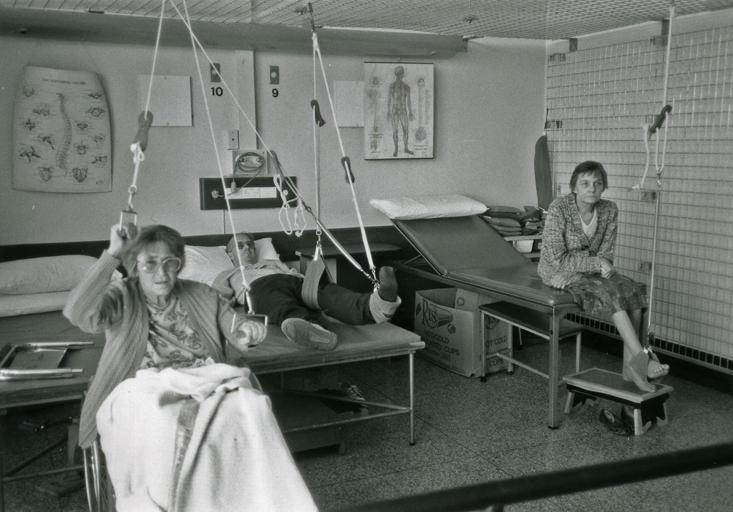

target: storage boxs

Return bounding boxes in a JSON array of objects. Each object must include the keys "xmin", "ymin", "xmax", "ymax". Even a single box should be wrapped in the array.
[{"xmin": 413, "ymin": 287, "xmax": 508, "ymax": 378}]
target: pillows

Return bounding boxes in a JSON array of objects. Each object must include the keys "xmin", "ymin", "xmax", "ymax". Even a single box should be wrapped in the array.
[
  {"xmin": 370, "ymin": 193, "xmax": 491, "ymax": 220},
  {"xmin": 0, "ymin": 254, "xmax": 123, "ymax": 295},
  {"xmin": 0, "ymin": 291, "xmax": 71, "ymax": 318},
  {"xmin": 177, "ymin": 237, "xmax": 280, "ymax": 288}
]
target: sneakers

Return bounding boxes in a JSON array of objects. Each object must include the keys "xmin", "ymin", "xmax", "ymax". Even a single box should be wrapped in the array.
[
  {"xmin": 342, "ymin": 384, "xmax": 367, "ymax": 402},
  {"xmin": 281, "ymin": 318, "xmax": 338, "ymax": 350}
]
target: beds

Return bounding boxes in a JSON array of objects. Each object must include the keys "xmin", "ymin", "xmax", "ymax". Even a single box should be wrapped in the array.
[
  {"xmin": 177, "ymin": 236, "xmax": 425, "ymax": 446},
  {"xmin": 368, "ymin": 193, "xmax": 576, "ymax": 429},
  {"xmin": 0, "ymin": 254, "xmax": 124, "ymax": 512}
]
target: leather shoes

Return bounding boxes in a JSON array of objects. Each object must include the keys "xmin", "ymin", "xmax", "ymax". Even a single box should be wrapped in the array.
[{"xmin": 600, "ymin": 406, "xmax": 634, "ymax": 436}]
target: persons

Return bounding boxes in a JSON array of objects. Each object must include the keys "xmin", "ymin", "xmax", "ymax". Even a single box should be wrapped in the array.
[
  {"xmin": 537, "ymin": 161, "xmax": 670, "ymax": 394},
  {"xmin": 211, "ymin": 234, "xmax": 398, "ymax": 352},
  {"xmin": 63, "ymin": 224, "xmax": 268, "ymax": 450}
]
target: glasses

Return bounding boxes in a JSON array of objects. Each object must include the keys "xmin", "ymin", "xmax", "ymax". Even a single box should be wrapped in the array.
[
  {"xmin": 238, "ymin": 240, "xmax": 255, "ymax": 249},
  {"xmin": 142, "ymin": 256, "xmax": 181, "ymax": 274}
]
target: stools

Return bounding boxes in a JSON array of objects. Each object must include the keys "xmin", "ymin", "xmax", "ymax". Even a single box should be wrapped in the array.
[
  {"xmin": 562, "ymin": 366, "xmax": 673, "ymax": 436},
  {"xmin": 479, "ymin": 301, "xmax": 586, "ymax": 383}
]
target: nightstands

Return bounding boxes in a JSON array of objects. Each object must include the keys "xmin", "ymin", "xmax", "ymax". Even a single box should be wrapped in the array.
[
  {"xmin": 295, "ymin": 242, "xmax": 403, "ymax": 284},
  {"xmin": 502, "ymin": 234, "xmax": 544, "ymax": 260}
]
